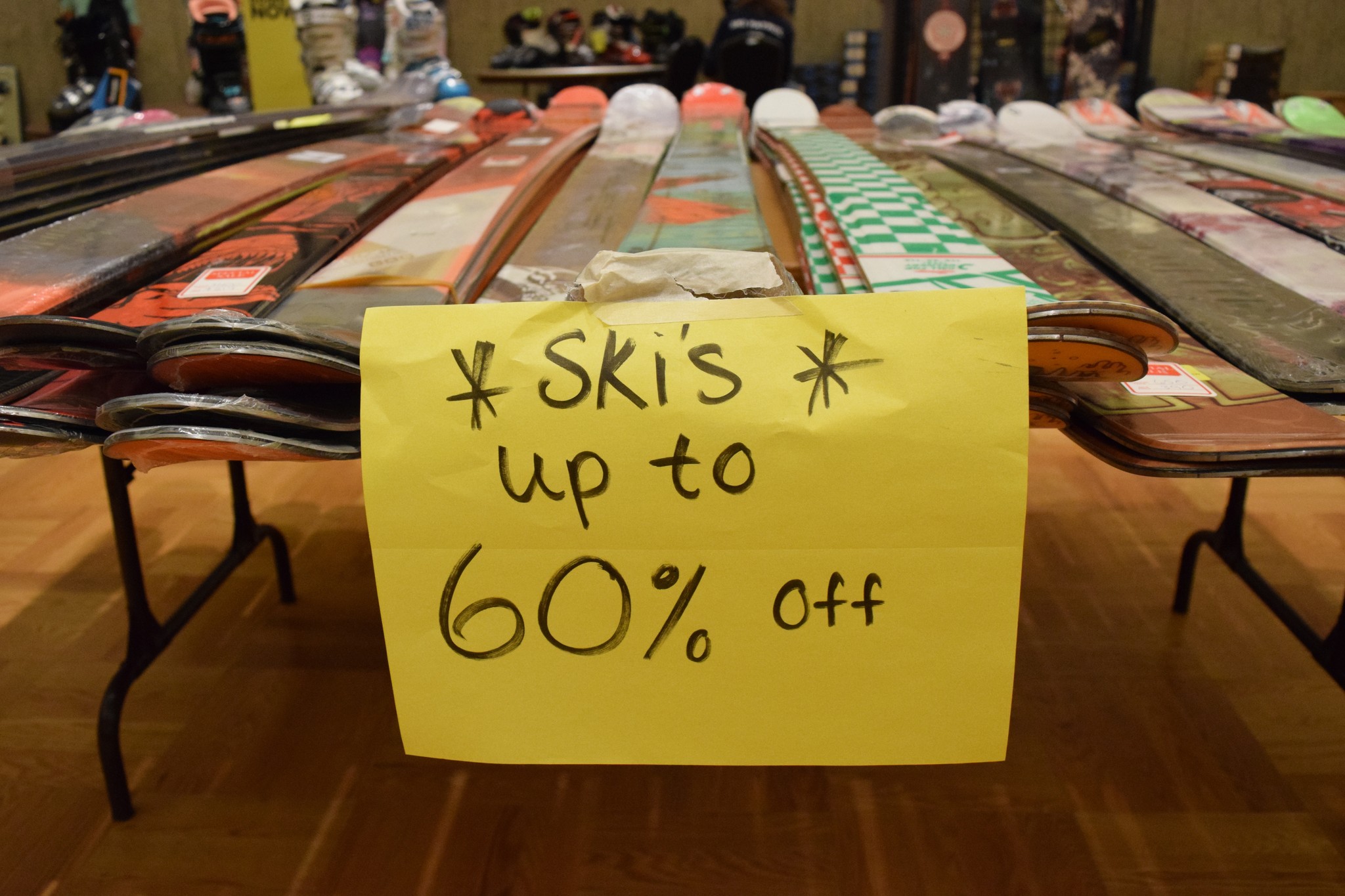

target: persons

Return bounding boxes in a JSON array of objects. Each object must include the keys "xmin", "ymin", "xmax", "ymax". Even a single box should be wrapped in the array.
[{"xmin": 703, "ymin": 2, "xmax": 797, "ymax": 111}]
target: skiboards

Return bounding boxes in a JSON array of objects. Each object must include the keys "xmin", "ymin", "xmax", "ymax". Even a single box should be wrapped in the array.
[{"xmin": 1, "ymin": 74, "xmax": 1345, "ymax": 495}]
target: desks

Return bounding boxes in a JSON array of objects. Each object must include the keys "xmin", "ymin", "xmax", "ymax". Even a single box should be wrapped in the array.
[{"xmin": 478, "ymin": 63, "xmax": 668, "ymax": 104}]
